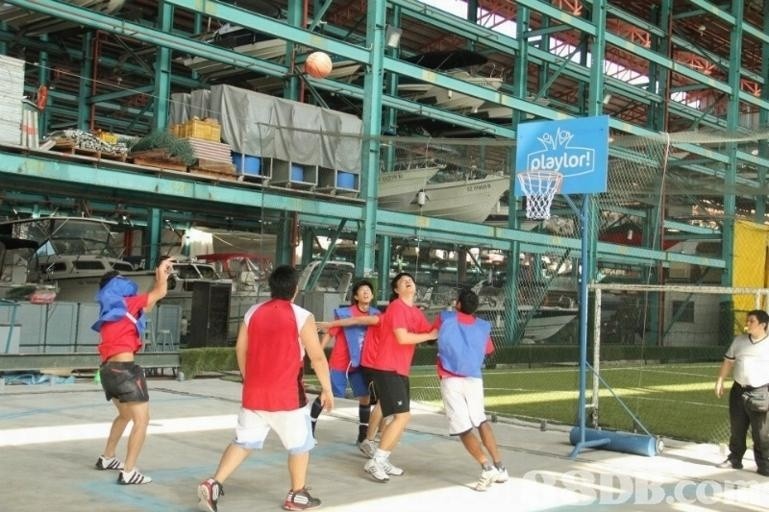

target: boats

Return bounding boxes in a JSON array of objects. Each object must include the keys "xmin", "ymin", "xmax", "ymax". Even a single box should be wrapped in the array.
[
  {"xmin": 179, "ymin": 19, "xmax": 548, "ymax": 125},
  {"xmin": 35, "ymin": 252, "xmax": 280, "ymax": 347},
  {"xmin": 405, "ymin": 299, "xmax": 581, "ymax": 346},
  {"xmin": 372, "ymin": 157, "xmax": 514, "ymax": 224}
]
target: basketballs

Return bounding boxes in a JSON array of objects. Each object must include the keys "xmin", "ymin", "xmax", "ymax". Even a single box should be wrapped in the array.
[{"xmin": 305, "ymin": 51, "xmax": 332, "ymax": 79}]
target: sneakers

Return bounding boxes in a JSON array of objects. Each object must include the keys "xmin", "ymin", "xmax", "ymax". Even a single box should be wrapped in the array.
[
  {"xmin": 284, "ymin": 489, "xmax": 321, "ymax": 510},
  {"xmin": 476, "ymin": 463, "xmax": 508, "ymax": 490},
  {"xmin": 96, "ymin": 455, "xmax": 125, "ymax": 470},
  {"xmin": 118, "ymin": 468, "xmax": 152, "ymax": 484},
  {"xmin": 197, "ymin": 479, "xmax": 220, "ymax": 512},
  {"xmin": 313, "ymin": 437, "xmax": 319, "ymax": 446},
  {"xmin": 358, "ymin": 432, "xmax": 404, "ymax": 480}
]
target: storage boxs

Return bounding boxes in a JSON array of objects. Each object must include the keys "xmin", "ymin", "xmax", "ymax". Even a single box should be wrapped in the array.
[{"xmin": 171, "ymin": 120, "xmax": 221, "ymax": 142}]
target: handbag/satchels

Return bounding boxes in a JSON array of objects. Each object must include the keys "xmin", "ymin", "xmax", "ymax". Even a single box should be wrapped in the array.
[{"xmin": 741, "ymin": 386, "xmax": 769, "ymax": 413}]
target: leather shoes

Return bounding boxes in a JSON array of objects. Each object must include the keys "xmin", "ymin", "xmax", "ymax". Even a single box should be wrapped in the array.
[{"xmin": 717, "ymin": 460, "xmax": 733, "ymax": 468}]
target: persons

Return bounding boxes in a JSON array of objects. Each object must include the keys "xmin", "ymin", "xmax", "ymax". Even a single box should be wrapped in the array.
[
  {"xmin": 364, "ymin": 273, "xmax": 438, "ymax": 483},
  {"xmin": 309, "ymin": 281, "xmax": 382, "ymax": 447},
  {"xmin": 196, "ymin": 266, "xmax": 336, "ymax": 512},
  {"xmin": 429, "ymin": 288, "xmax": 509, "ymax": 491},
  {"xmin": 714, "ymin": 310, "xmax": 768, "ymax": 477},
  {"xmin": 317, "ymin": 293, "xmax": 399, "ymax": 460},
  {"xmin": 96, "ymin": 256, "xmax": 178, "ymax": 485}
]
